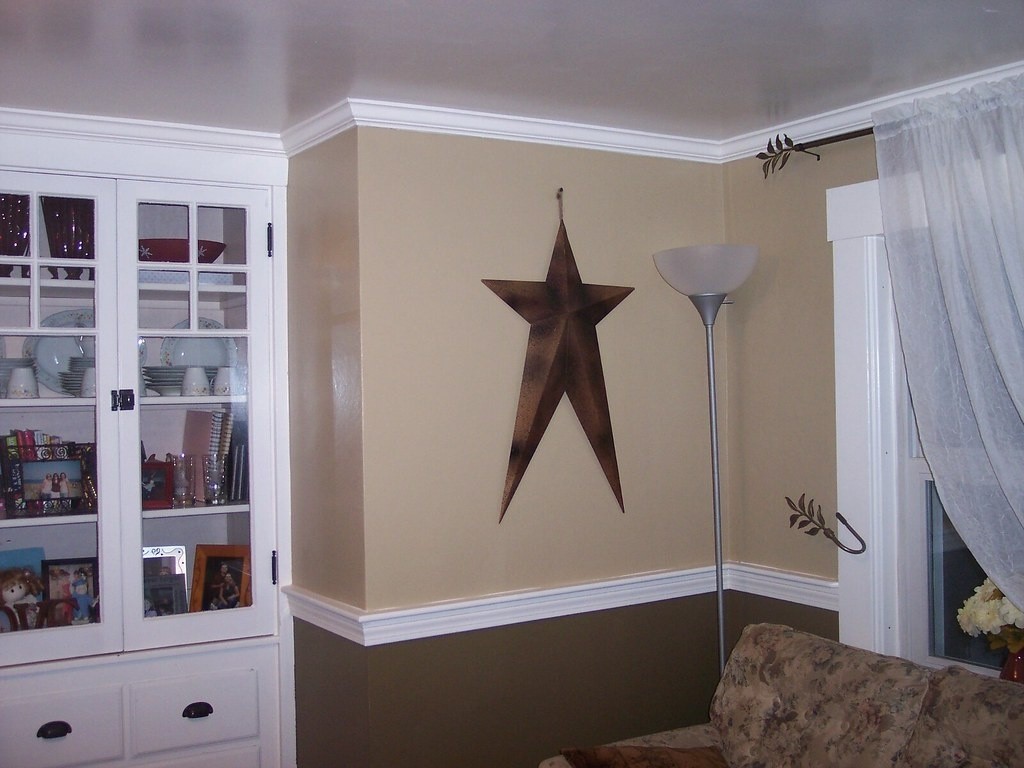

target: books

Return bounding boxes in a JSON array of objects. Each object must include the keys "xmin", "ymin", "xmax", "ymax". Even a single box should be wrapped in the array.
[{"xmin": 182, "ymin": 409, "xmax": 233, "ymax": 503}]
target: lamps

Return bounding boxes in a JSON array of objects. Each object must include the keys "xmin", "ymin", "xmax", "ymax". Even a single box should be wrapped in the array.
[{"xmin": 653, "ymin": 243, "xmax": 760, "ymax": 680}]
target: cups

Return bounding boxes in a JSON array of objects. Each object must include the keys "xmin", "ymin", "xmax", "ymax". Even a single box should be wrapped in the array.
[
  {"xmin": 79, "ymin": 367, "xmax": 96, "ymax": 398},
  {"xmin": 170, "ymin": 455, "xmax": 195, "ymax": 508},
  {"xmin": 6, "ymin": 368, "xmax": 40, "ymax": 399},
  {"xmin": 209, "ymin": 367, "xmax": 242, "ymax": 396},
  {"xmin": 180, "ymin": 368, "xmax": 209, "ymax": 396},
  {"xmin": 202, "ymin": 454, "xmax": 228, "ymax": 506}
]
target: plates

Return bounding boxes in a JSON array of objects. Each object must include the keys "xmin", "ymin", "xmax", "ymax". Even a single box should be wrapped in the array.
[
  {"xmin": 22, "ymin": 309, "xmax": 148, "ymax": 395},
  {"xmin": 159, "ymin": 317, "xmax": 239, "ymax": 369},
  {"xmin": 141, "ymin": 365, "xmax": 228, "ymax": 396},
  {"xmin": 0, "ymin": 357, "xmax": 38, "ymax": 399},
  {"xmin": 57, "ymin": 356, "xmax": 95, "ymax": 398}
]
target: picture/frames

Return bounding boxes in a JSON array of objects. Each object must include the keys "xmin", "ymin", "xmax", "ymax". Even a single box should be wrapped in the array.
[
  {"xmin": 143, "ymin": 545, "xmax": 187, "ymax": 600},
  {"xmin": 144, "ymin": 574, "xmax": 188, "ymax": 616},
  {"xmin": 141, "ymin": 463, "xmax": 172, "ymax": 511},
  {"xmin": 41, "ymin": 557, "xmax": 100, "ymax": 626},
  {"xmin": 189, "ymin": 544, "xmax": 253, "ymax": 612}
]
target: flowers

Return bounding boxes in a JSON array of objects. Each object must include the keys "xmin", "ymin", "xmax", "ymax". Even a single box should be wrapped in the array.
[{"xmin": 957, "ymin": 576, "xmax": 1024, "ymax": 653}]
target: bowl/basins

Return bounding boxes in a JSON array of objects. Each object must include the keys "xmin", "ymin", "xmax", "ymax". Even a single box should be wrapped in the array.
[{"xmin": 139, "ymin": 238, "xmax": 228, "ymax": 264}]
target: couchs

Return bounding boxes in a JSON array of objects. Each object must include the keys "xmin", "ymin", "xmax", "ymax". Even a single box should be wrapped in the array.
[{"xmin": 539, "ymin": 623, "xmax": 1024, "ymax": 768}]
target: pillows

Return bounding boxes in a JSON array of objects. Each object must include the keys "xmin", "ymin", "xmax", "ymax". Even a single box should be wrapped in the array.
[{"xmin": 560, "ymin": 746, "xmax": 730, "ymax": 768}]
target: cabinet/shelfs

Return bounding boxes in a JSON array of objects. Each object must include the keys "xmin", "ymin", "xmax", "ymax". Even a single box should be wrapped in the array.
[{"xmin": 1, "ymin": 165, "xmax": 280, "ymax": 768}]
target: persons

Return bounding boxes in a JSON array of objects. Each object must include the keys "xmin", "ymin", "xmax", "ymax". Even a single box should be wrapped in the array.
[
  {"xmin": 57, "ymin": 570, "xmax": 84, "ymax": 599},
  {"xmin": 39, "ymin": 472, "xmax": 70, "ymax": 499},
  {"xmin": 203, "ymin": 561, "xmax": 240, "ymax": 610}
]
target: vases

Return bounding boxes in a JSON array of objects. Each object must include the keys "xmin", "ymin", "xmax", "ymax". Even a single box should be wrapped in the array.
[{"xmin": 999, "ymin": 648, "xmax": 1024, "ymax": 683}]
